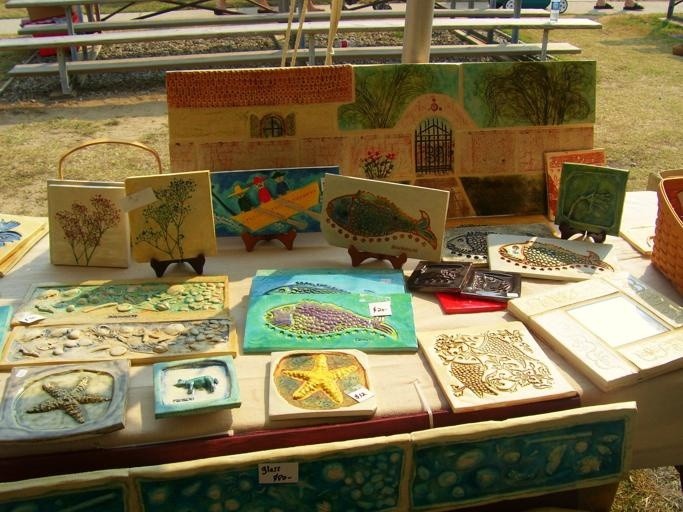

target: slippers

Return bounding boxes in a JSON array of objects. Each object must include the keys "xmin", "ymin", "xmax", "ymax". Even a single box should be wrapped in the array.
[{"xmin": 594, "ymin": 3, "xmax": 644, "ymax": 10}]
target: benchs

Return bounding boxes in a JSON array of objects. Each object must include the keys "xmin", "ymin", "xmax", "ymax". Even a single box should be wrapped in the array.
[{"xmin": 0, "ymin": 7, "xmax": 603, "ymax": 98}]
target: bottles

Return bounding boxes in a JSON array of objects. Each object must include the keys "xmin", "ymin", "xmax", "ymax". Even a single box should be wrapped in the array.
[{"xmin": 550, "ymin": 0, "xmax": 560, "ymax": 21}]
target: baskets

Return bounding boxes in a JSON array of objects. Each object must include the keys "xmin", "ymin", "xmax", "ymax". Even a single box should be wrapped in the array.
[{"xmin": 651, "ymin": 176, "xmax": 683, "ymax": 297}]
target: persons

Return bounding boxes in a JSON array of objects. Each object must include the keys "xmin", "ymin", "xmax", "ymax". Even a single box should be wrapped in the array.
[
  {"xmin": 594, "ymin": 0, "xmax": 645, "ymax": 10},
  {"xmin": 212, "ymin": 0, "xmax": 325, "ymax": 15}
]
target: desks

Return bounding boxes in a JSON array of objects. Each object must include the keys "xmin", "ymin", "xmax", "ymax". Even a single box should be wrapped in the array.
[{"xmin": 0, "ymin": 190, "xmax": 682, "ymax": 460}]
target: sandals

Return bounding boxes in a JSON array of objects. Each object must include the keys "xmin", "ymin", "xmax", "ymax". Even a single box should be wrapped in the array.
[{"xmin": 214, "ymin": 9, "xmax": 277, "ymax": 15}]
